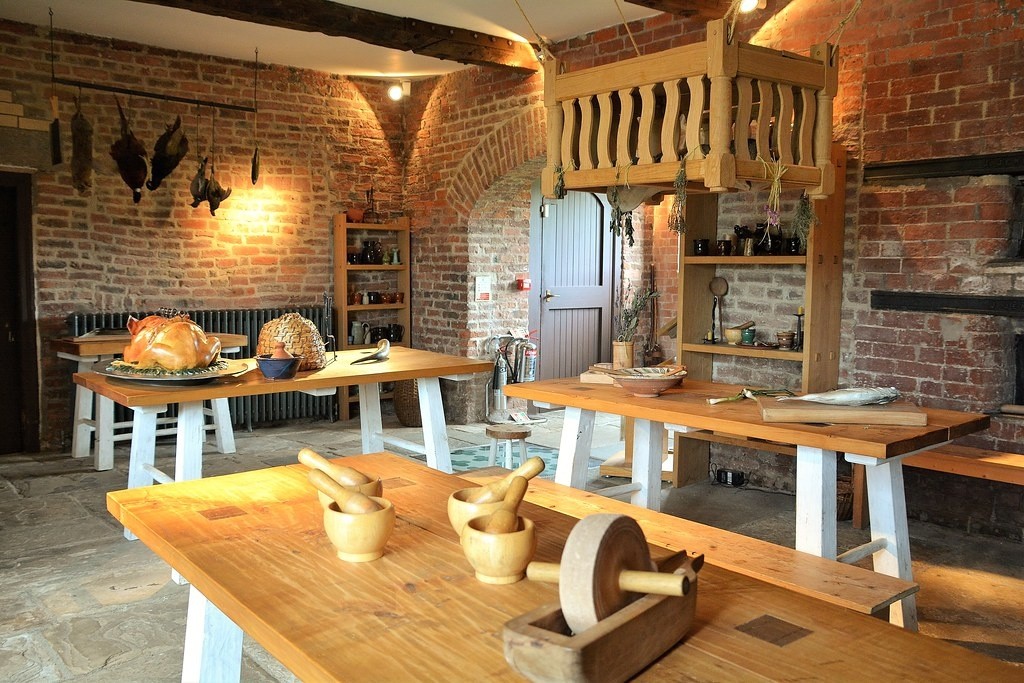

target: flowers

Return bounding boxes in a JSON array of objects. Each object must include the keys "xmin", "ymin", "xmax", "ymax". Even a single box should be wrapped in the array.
[{"xmin": 757, "ymin": 155, "xmax": 790, "ymax": 246}]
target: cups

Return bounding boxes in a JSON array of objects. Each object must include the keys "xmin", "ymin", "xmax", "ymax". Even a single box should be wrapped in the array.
[
  {"xmin": 786, "ymin": 238, "xmax": 800, "ymax": 256},
  {"xmin": 693, "ymin": 239, "xmax": 710, "ymax": 256},
  {"xmin": 717, "ymin": 240, "xmax": 731, "ymax": 256}
]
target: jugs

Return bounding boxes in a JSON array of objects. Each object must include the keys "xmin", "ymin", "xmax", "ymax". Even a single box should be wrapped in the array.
[
  {"xmin": 352, "ymin": 321, "xmax": 370, "ymax": 344},
  {"xmin": 363, "ymin": 241, "xmax": 382, "ymax": 264}
]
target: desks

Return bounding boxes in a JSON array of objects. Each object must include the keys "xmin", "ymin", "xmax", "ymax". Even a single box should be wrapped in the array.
[{"xmin": 50, "ymin": 332, "xmax": 247, "ymax": 472}]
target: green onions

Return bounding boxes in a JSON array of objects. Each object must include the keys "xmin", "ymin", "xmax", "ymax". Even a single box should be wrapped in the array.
[{"xmin": 706, "ymin": 388, "xmax": 765, "ymax": 405}]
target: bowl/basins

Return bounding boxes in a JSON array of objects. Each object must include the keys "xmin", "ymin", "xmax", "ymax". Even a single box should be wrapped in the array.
[
  {"xmin": 607, "ymin": 367, "xmax": 688, "ymax": 397},
  {"xmin": 253, "ymin": 354, "xmax": 304, "ymax": 379}
]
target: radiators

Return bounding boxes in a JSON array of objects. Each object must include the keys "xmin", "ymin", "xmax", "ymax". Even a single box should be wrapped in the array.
[{"xmin": 66, "ymin": 302, "xmax": 335, "ymax": 441}]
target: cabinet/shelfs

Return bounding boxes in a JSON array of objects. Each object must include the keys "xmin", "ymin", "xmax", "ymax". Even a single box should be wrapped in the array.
[
  {"xmin": 674, "ymin": 143, "xmax": 847, "ymax": 494},
  {"xmin": 334, "ymin": 213, "xmax": 411, "ymax": 421}
]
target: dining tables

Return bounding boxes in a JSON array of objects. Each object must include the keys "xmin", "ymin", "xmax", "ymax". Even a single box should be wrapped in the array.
[
  {"xmin": 502, "ymin": 373, "xmax": 991, "ymax": 632},
  {"xmin": 106, "ymin": 452, "xmax": 1024, "ymax": 683},
  {"xmin": 73, "ymin": 347, "xmax": 494, "ymax": 586}
]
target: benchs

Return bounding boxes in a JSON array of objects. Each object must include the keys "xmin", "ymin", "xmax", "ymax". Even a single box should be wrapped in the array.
[
  {"xmin": 850, "ymin": 444, "xmax": 1024, "ymax": 529},
  {"xmin": 450, "ymin": 466, "xmax": 921, "ymax": 614}
]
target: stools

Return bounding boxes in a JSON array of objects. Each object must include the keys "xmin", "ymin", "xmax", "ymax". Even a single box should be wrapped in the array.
[{"xmin": 486, "ymin": 424, "xmax": 531, "ymax": 470}]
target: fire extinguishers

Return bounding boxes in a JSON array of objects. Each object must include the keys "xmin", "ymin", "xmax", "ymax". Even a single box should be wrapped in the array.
[
  {"xmin": 492, "ymin": 344, "xmax": 513, "ymax": 410},
  {"xmin": 505, "ymin": 329, "xmax": 539, "ymax": 385}
]
